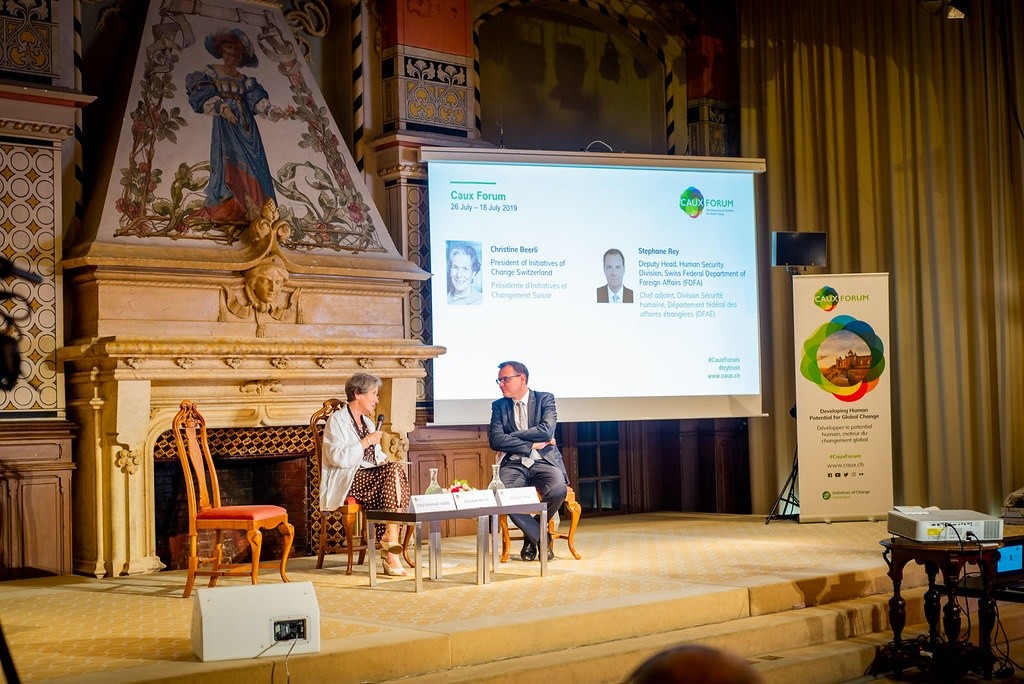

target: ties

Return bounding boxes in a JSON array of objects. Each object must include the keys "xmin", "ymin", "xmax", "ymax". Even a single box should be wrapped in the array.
[
  {"xmin": 613, "ymin": 294, "xmax": 619, "ymax": 303},
  {"xmin": 516, "ymin": 401, "xmax": 535, "ymax": 468}
]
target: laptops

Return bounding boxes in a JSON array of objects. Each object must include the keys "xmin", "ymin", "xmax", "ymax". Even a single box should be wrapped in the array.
[{"xmin": 996, "ymin": 538, "xmax": 1024, "ymax": 593}]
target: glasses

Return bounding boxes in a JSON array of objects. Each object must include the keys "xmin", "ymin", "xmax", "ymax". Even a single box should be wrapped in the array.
[{"xmin": 495, "ymin": 374, "xmax": 521, "ymax": 384}]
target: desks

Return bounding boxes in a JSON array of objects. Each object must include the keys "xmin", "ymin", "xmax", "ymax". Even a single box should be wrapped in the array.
[
  {"xmin": 366, "ymin": 502, "xmax": 547, "ymax": 592},
  {"xmin": 880, "ymin": 536, "xmax": 1006, "ymax": 684}
]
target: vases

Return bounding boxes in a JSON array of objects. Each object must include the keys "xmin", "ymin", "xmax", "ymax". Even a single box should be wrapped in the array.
[
  {"xmin": 487, "ymin": 464, "xmax": 507, "ymax": 500},
  {"xmin": 426, "ymin": 468, "xmax": 446, "ymax": 507}
]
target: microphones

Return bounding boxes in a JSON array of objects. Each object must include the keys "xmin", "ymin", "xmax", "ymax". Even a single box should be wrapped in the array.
[{"xmin": 375, "ymin": 414, "xmax": 385, "ymax": 431}]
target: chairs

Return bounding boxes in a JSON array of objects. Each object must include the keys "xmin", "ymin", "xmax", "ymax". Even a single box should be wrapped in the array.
[
  {"xmin": 495, "ymin": 450, "xmax": 582, "ymax": 563},
  {"xmin": 172, "ymin": 399, "xmax": 295, "ymax": 601},
  {"xmin": 310, "ymin": 399, "xmax": 424, "ymax": 575}
]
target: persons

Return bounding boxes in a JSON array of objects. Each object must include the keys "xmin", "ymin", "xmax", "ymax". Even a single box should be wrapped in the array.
[
  {"xmin": 489, "ymin": 360, "xmax": 570, "ymax": 561},
  {"xmin": 597, "ymin": 249, "xmax": 633, "ymax": 303},
  {"xmin": 318, "ymin": 372, "xmax": 410, "ymax": 576},
  {"xmin": 634, "ymin": 644, "xmax": 766, "ymax": 684},
  {"xmin": 447, "ymin": 244, "xmax": 482, "ymax": 304}
]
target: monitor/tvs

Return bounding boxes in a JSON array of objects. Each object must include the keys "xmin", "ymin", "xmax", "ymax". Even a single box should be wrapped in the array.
[{"xmin": 771, "ymin": 231, "xmax": 827, "ymax": 267}]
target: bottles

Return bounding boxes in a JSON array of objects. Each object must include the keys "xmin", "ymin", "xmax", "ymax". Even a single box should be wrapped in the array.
[
  {"xmin": 424, "ymin": 468, "xmax": 443, "ymax": 495},
  {"xmin": 487, "ymin": 465, "xmax": 505, "ymax": 492}
]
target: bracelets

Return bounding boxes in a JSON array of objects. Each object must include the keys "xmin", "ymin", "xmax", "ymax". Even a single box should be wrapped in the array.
[{"xmin": 366, "ymin": 436, "xmax": 370, "ymax": 445}]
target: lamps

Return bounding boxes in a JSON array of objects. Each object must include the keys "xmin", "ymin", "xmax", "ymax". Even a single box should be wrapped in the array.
[
  {"xmin": 189, "ymin": 581, "xmax": 321, "ymax": 664},
  {"xmin": 916, "ymin": 0, "xmax": 973, "ymax": 21}
]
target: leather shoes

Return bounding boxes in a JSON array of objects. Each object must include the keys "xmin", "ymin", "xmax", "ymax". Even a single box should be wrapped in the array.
[
  {"xmin": 521, "ymin": 533, "xmax": 537, "ymax": 561},
  {"xmin": 538, "ymin": 532, "xmax": 554, "ymax": 561}
]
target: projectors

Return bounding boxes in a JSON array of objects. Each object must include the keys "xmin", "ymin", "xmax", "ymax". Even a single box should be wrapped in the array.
[{"xmin": 888, "ymin": 509, "xmax": 1004, "ymax": 542}]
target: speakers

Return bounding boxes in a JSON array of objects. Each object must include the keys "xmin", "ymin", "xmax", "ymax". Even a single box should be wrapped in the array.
[{"xmin": 190, "ymin": 581, "xmax": 320, "ymax": 664}]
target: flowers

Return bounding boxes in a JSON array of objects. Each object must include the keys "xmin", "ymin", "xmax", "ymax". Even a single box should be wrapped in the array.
[{"xmin": 449, "ymin": 478, "xmax": 475, "ymax": 495}]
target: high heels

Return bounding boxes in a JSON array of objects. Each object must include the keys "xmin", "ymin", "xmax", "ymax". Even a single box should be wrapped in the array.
[
  {"xmin": 382, "ymin": 560, "xmax": 407, "ymax": 576},
  {"xmin": 380, "ymin": 541, "xmax": 403, "ymax": 559}
]
